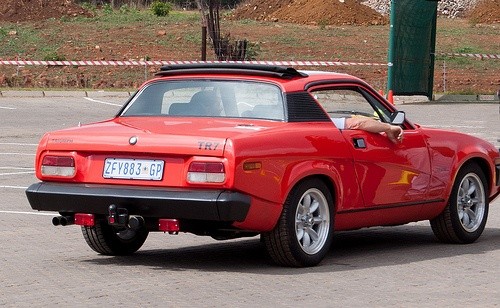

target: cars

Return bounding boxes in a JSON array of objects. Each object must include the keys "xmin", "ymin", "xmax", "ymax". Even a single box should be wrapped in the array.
[{"xmin": 26, "ymin": 63, "xmax": 496, "ymax": 269}]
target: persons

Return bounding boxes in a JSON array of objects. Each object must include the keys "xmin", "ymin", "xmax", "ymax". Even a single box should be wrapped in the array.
[
  {"xmin": 327, "ymin": 114, "xmax": 404, "ymax": 145},
  {"xmin": 190, "ymin": 91, "xmax": 223, "ymax": 118}
]
